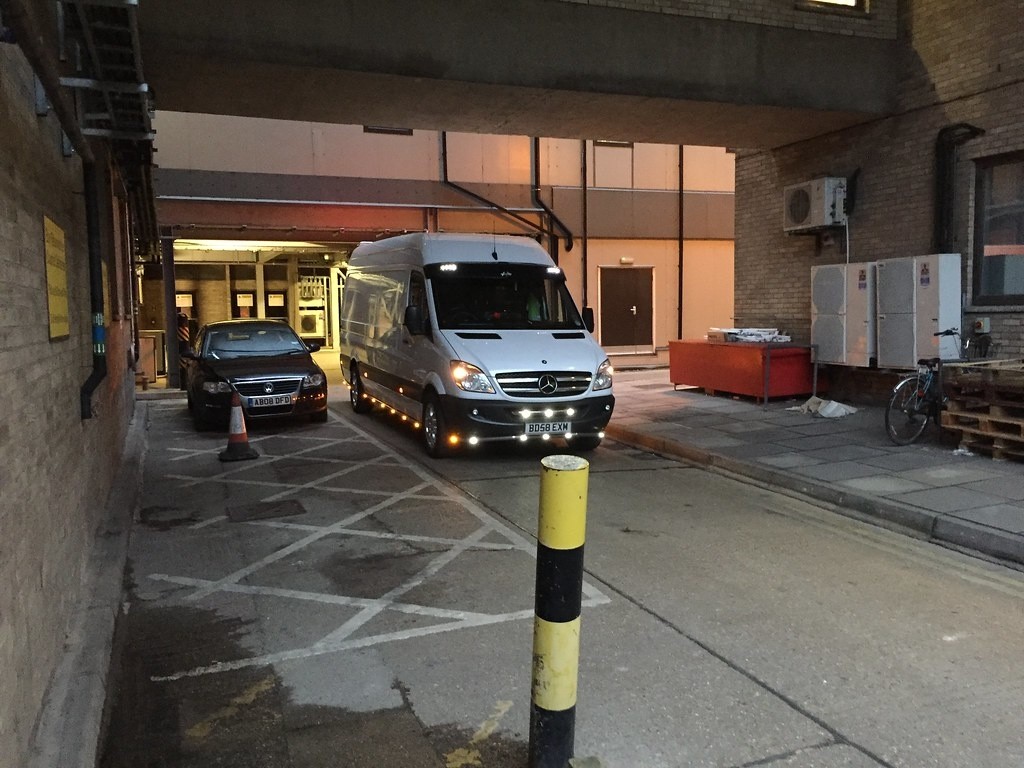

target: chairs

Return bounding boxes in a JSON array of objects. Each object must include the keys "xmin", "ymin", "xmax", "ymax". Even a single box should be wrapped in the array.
[
  {"xmin": 427, "ymin": 293, "xmax": 462, "ymax": 329},
  {"xmin": 481, "ymin": 288, "xmax": 527, "ymax": 323}
]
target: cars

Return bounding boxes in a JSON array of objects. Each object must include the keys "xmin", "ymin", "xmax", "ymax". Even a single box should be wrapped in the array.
[{"xmin": 182, "ymin": 319, "xmax": 328, "ymax": 437}]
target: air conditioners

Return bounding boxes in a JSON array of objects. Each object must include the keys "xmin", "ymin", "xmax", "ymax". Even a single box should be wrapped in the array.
[
  {"xmin": 783, "ymin": 177, "xmax": 846, "ymax": 233},
  {"xmin": 876, "ymin": 253, "xmax": 962, "ymax": 371},
  {"xmin": 296, "ymin": 282, "xmax": 325, "ymax": 307},
  {"xmin": 297, "ymin": 310, "xmax": 326, "ymax": 339},
  {"xmin": 810, "ymin": 262, "xmax": 876, "ymax": 368}
]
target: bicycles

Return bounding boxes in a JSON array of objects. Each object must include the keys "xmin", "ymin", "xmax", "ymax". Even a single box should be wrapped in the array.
[{"xmin": 881, "ymin": 331, "xmax": 1004, "ymax": 447}]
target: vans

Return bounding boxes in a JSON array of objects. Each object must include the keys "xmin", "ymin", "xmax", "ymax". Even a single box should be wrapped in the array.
[{"xmin": 340, "ymin": 232, "xmax": 618, "ymax": 458}]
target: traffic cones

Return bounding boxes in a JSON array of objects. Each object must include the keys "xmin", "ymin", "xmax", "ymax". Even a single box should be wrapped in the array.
[{"xmin": 217, "ymin": 391, "xmax": 260, "ymax": 462}]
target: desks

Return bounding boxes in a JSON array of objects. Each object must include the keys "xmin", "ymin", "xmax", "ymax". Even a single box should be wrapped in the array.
[{"xmin": 668, "ymin": 339, "xmax": 829, "ymax": 405}]
